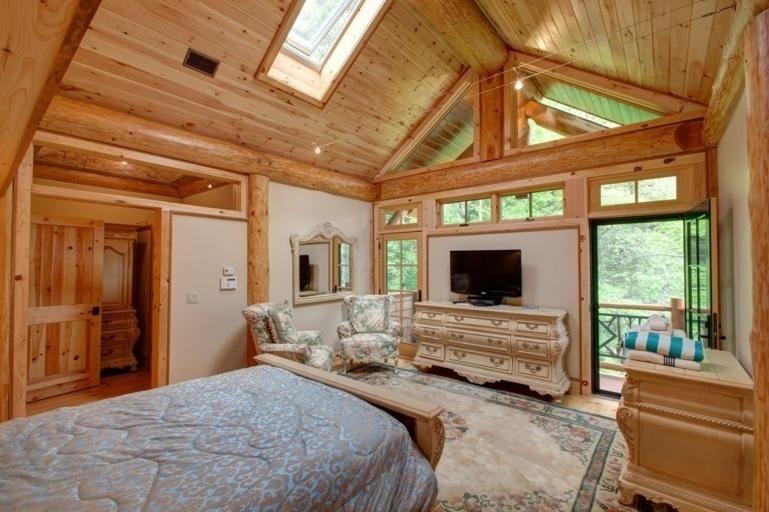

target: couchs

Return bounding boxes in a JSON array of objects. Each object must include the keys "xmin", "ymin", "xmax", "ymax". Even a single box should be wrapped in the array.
[
  {"xmin": 239, "ymin": 301, "xmax": 333, "ymax": 375},
  {"xmin": 337, "ymin": 295, "xmax": 406, "ymax": 376}
]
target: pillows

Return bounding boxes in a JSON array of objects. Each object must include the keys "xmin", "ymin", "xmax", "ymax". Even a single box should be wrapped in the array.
[
  {"xmin": 266, "ymin": 301, "xmax": 293, "ymax": 344},
  {"xmin": 283, "ymin": 329, "xmax": 321, "ymax": 348}
]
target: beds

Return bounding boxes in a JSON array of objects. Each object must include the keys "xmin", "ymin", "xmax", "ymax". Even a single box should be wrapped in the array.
[{"xmin": 2, "ymin": 353, "xmax": 444, "ymax": 510}]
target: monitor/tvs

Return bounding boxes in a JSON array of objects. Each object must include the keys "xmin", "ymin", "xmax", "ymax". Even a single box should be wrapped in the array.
[
  {"xmin": 449, "ymin": 250, "xmax": 521, "ymax": 305},
  {"xmin": 300, "ymin": 255, "xmax": 310, "ymax": 289}
]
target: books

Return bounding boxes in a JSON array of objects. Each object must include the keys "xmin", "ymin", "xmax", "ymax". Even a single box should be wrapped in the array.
[{"xmin": 523, "ymin": 305, "xmax": 538, "ymax": 312}]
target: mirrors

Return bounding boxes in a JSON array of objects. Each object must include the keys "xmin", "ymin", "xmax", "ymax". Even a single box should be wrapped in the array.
[{"xmin": 289, "ymin": 220, "xmax": 359, "ymax": 306}]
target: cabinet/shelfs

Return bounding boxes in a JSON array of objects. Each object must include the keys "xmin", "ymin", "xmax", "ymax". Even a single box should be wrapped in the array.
[
  {"xmin": 413, "ymin": 302, "xmax": 569, "ymax": 404},
  {"xmin": 102, "ymin": 231, "xmax": 140, "ymax": 370}
]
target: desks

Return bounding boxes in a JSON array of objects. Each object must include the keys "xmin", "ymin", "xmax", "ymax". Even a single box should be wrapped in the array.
[{"xmin": 619, "ymin": 343, "xmax": 755, "ymax": 512}]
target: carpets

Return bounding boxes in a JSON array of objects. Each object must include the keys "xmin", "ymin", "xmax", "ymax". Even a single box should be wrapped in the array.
[{"xmin": 346, "ymin": 361, "xmax": 629, "ymax": 511}]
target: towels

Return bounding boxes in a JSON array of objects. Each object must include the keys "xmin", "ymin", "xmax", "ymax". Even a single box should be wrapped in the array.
[{"xmin": 621, "ymin": 315, "xmax": 706, "ymax": 372}]
